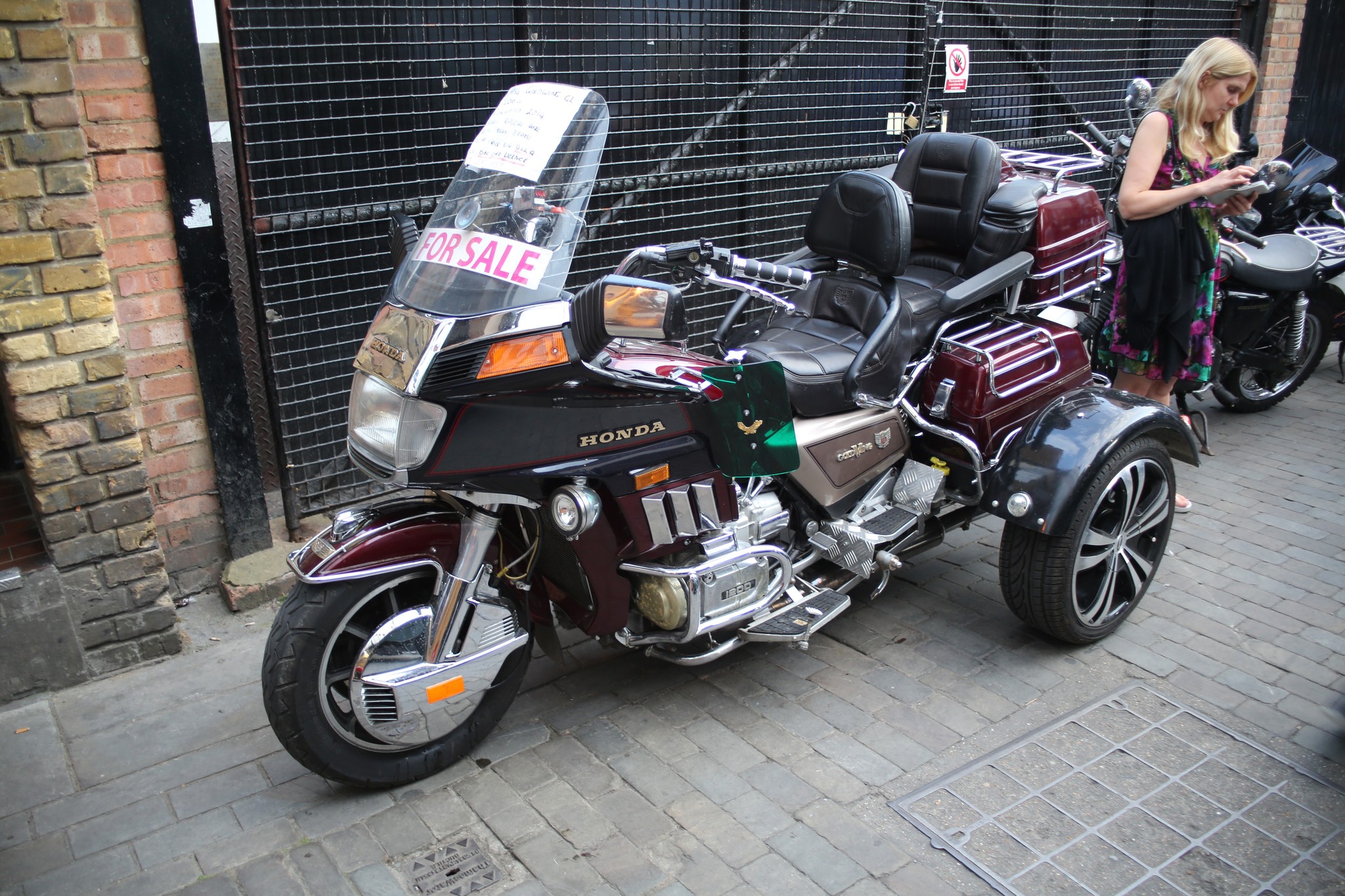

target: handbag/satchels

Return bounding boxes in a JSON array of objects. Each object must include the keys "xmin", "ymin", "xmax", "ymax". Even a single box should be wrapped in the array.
[{"xmin": 1104, "ymin": 108, "xmax": 1183, "ymax": 237}]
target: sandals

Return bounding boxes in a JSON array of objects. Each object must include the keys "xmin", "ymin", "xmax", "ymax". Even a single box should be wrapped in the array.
[{"xmin": 1175, "ymin": 496, "xmax": 1192, "ymax": 512}]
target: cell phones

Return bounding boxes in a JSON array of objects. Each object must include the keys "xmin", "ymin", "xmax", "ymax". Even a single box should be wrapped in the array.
[{"xmin": 1238, "ymin": 180, "xmax": 1269, "ymax": 198}]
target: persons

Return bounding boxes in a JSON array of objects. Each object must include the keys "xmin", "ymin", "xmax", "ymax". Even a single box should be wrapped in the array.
[{"xmin": 1098, "ymin": 37, "xmax": 1260, "ymax": 515}]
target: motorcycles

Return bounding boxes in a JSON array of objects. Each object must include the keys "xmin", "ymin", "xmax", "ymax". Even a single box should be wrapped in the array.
[
  {"xmin": 258, "ymin": 80, "xmax": 1208, "ymax": 796},
  {"xmin": 1044, "ymin": 76, "xmax": 1345, "ymax": 417}
]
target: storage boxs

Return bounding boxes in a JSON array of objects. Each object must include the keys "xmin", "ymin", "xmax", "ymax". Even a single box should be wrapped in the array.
[
  {"xmin": 910, "ymin": 313, "xmax": 1094, "ymax": 465},
  {"xmin": 997, "ymin": 158, "xmax": 1111, "ymax": 301}
]
